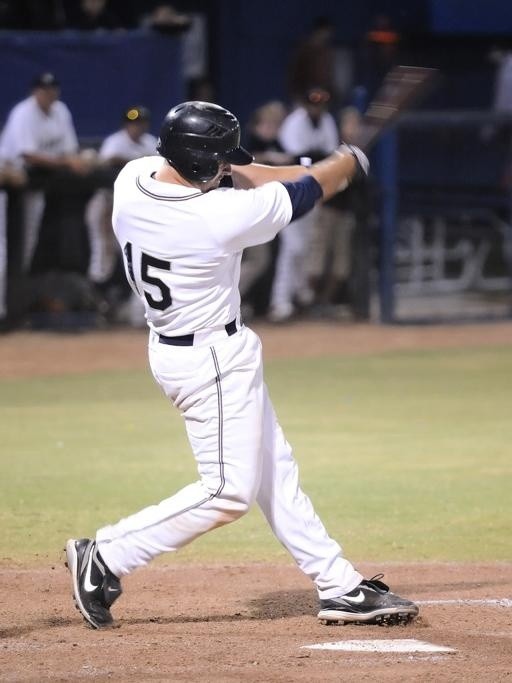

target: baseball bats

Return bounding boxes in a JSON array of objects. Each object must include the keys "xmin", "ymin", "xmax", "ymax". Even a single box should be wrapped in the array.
[{"xmin": 353, "ymin": 65, "xmax": 438, "ymax": 152}]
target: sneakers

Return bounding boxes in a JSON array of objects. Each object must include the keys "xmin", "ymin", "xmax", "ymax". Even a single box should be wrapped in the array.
[
  {"xmin": 317, "ymin": 579, "xmax": 419, "ymax": 626},
  {"xmin": 64, "ymin": 538, "xmax": 123, "ymax": 628}
]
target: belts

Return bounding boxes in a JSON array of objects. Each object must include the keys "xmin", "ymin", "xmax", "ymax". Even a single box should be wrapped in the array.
[{"xmin": 159, "ymin": 318, "xmax": 237, "ymax": 346}]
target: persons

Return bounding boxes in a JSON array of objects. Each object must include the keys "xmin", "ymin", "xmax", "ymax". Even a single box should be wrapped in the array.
[{"xmin": 62, "ymin": 100, "xmax": 420, "ymax": 631}]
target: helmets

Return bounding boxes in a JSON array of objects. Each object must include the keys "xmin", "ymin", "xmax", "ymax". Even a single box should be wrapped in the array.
[{"xmin": 156, "ymin": 101, "xmax": 255, "ymax": 183}]
